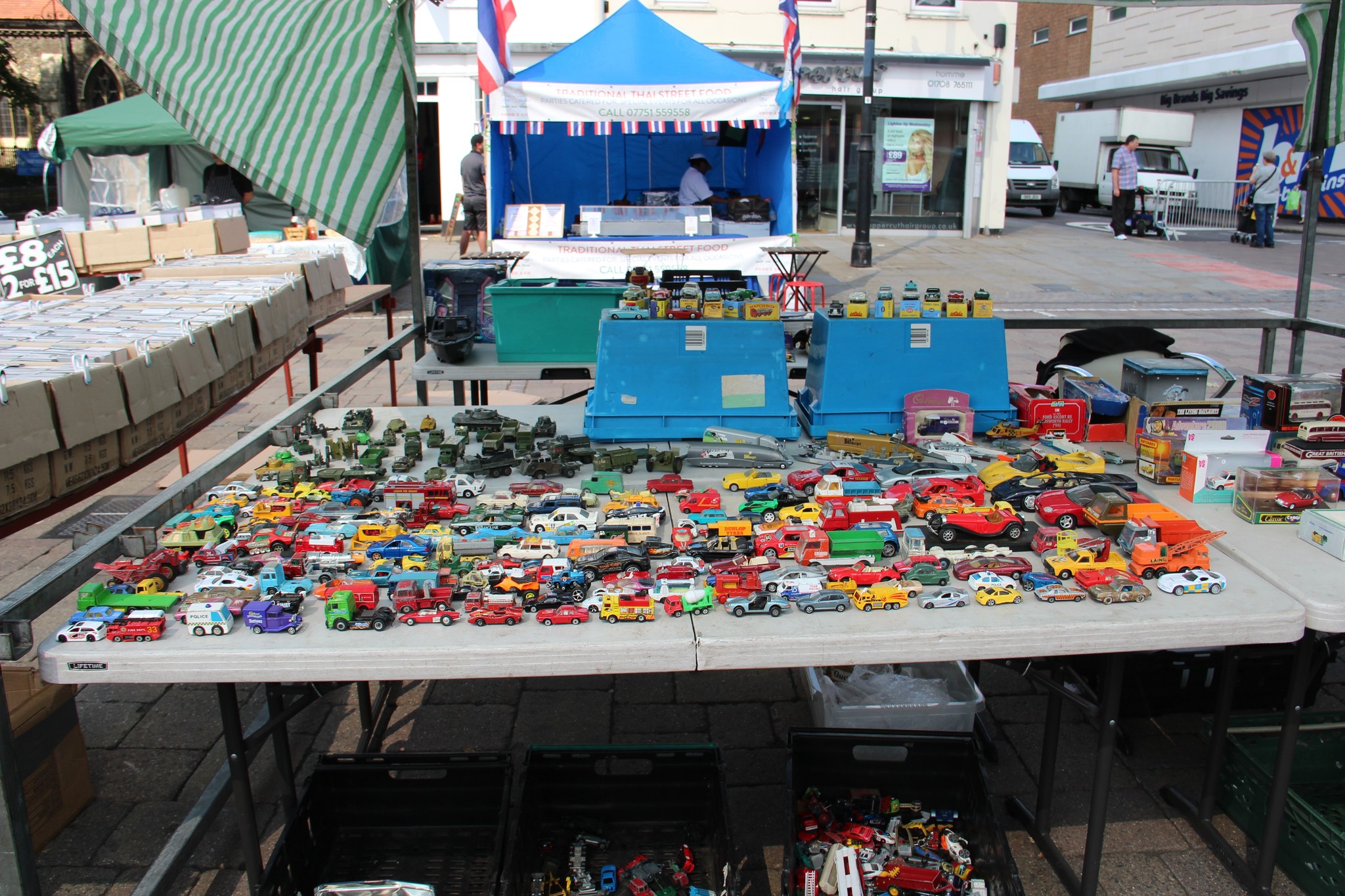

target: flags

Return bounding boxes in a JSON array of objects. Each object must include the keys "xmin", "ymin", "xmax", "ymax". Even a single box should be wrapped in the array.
[
  {"xmin": 775, "ymin": 0, "xmax": 802, "ymax": 127},
  {"xmin": 477, "ymin": 0, "xmax": 516, "ymax": 93}
]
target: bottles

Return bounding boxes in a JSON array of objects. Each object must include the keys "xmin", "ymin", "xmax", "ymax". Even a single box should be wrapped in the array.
[
  {"xmin": 290, "ymin": 215, "xmax": 305, "ymax": 227},
  {"xmin": 307, "ymin": 218, "xmax": 319, "ymax": 241}
]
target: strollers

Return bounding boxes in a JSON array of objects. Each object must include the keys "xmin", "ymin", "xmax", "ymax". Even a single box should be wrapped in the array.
[{"xmin": 1231, "ymin": 193, "xmax": 1266, "ymax": 245}]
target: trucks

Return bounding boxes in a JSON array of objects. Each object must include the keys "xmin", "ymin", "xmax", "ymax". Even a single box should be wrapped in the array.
[
  {"xmin": 358, "ymin": 428, "xmax": 678, "ymax": 480},
  {"xmin": 1051, "ymin": 106, "xmax": 1198, "ymax": 220},
  {"xmin": 918, "ymin": 413, "xmax": 959, "ymax": 436}
]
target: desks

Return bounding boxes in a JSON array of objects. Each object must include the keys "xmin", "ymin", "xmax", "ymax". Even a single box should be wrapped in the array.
[
  {"xmin": 180, "ymin": 277, "xmax": 403, "ymax": 508},
  {"xmin": 47, "ymin": 400, "xmax": 1301, "ymax": 896},
  {"xmin": 1074, "ymin": 425, "xmax": 1345, "ymax": 896},
  {"xmin": 761, "ymin": 243, "xmax": 827, "ymax": 311}
]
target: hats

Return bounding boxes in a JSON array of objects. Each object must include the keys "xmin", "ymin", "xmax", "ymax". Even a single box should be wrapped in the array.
[{"xmin": 688, "ymin": 154, "xmax": 713, "ymax": 172}]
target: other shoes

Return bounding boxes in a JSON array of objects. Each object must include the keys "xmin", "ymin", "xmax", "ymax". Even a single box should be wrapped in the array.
[
  {"xmin": 1265, "ymin": 242, "xmax": 1274, "ymax": 249},
  {"xmin": 1297, "ymin": 221, "xmax": 1304, "ymax": 224},
  {"xmin": 1249, "ymin": 241, "xmax": 1264, "ymax": 249}
]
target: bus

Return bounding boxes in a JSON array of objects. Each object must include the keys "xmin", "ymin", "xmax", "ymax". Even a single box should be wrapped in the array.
[
  {"xmin": 1289, "ymin": 398, "xmax": 1332, "ymax": 419},
  {"xmin": 1297, "ymin": 421, "xmax": 1345, "ymax": 442}
]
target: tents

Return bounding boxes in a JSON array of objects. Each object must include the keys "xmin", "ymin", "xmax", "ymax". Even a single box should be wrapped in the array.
[
  {"xmin": 40, "ymin": 90, "xmax": 410, "ymax": 316},
  {"xmin": 482, "ymin": 0, "xmax": 799, "ymax": 256}
]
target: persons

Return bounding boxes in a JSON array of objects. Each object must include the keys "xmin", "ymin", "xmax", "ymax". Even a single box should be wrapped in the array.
[
  {"xmin": 423, "ymin": 144, "xmax": 441, "ymax": 224},
  {"xmin": 203, "ymin": 153, "xmax": 253, "ymax": 220},
  {"xmin": 678, "ymin": 153, "xmax": 728, "ymax": 205},
  {"xmin": 1249, "ymin": 151, "xmax": 1280, "ymax": 248},
  {"xmin": 1108, "ymin": 135, "xmax": 1140, "ymax": 240},
  {"xmin": 460, "ymin": 135, "xmax": 486, "ymax": 256}
]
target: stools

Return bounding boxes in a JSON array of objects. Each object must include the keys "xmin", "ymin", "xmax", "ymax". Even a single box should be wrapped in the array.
[
  {"xmin": 783, "ymin": 280, "xmax": 825, "ymax": 315},
  {"xmin": 769, "ymin": 272, "xmax": 806, "ymax": 310}
]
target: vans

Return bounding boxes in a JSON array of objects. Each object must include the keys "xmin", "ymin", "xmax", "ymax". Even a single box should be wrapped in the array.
[{"xmin": 1006, "ymin": 118, "xmax": 1059, "ymax": 217}]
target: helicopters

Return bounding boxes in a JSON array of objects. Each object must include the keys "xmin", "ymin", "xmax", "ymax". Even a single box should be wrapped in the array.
[{"xmin": 981, "ymin": 414, "xmax": 1046, "ymax": 439}]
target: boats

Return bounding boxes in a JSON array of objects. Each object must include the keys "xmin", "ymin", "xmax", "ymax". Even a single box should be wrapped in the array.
[{"xmin": 428, "ymin": 314, "xmax": 476, "ymax": 363}]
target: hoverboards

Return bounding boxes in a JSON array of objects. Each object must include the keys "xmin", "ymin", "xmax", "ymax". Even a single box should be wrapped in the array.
[{"xmin": 1124, "ymin": 186, "xmax": 1164, "ymax": 237}]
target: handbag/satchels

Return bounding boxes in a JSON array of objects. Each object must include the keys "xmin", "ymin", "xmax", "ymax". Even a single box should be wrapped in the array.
[
  {"xmin": 1247, "ymin": 188, "xmax": 1256, "ymax": 204},
  {"xmin": 1285, "ymin": 188, "xmax": 1301, "ymax": 210}
]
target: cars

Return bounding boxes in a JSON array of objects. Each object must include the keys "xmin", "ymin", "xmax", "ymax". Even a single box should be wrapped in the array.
[
  {"xmin": 59, "ymin": 442, "xmax": 1227, "ymax": 642},
  {"xmin": 532, "ymin": 781, "xmax": 986, "ymax": 896},
  {"xmin": 1274, "ymin": 486, "xmax": 1323, "ymax": 510},
  {"xmin": 605, "ymin": 282, "xmax": 989, "ymax": 324},
  {"xmin": 1205, "ymin": 470, "xmax": 1236, "ymax": 490}
]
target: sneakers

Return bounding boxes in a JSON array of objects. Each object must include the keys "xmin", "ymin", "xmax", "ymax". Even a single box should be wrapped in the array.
[
  {"xmin": 1107, "ymin": 225, "xmax": 1114, "ymax": 234},
  {"xmin": 1113, "ymin": 232, "xmax": 1127, "ymax": 240}
]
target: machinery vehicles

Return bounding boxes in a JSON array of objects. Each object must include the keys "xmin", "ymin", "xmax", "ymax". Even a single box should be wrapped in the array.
[{"xmin": 297, "ymin": 406, "xmax": 557, "ymax": 436}]
